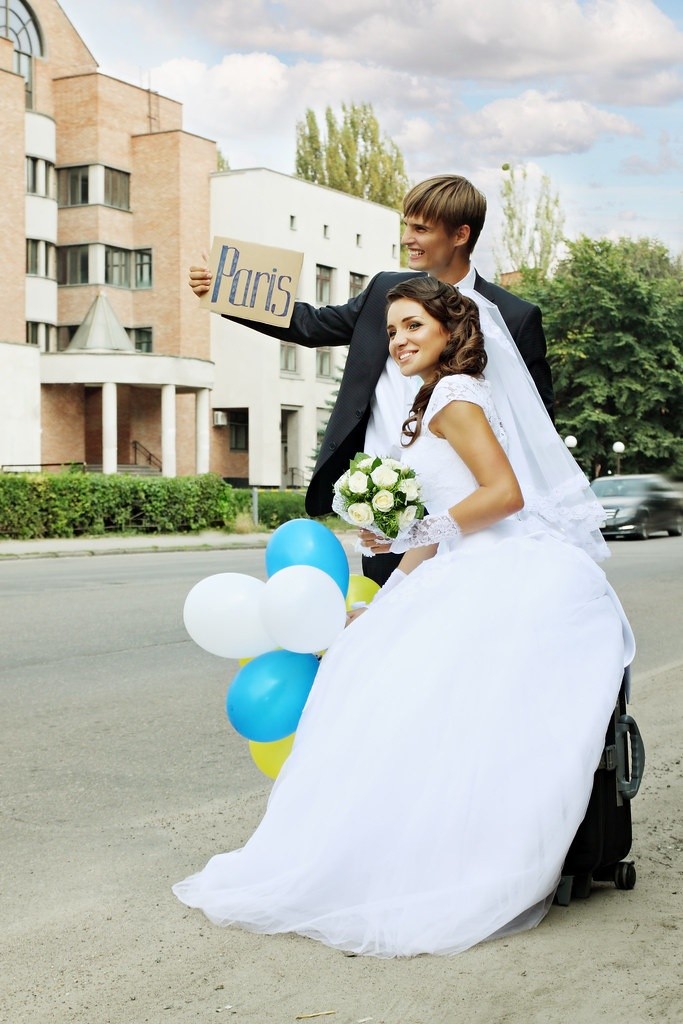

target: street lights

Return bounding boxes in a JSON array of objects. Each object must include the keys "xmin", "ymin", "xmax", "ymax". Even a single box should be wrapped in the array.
[
  {"xmin": 564, "ymin": 435, "xmax": 577, "ymax": 457},
  {"xmin": 613, "ymin": 441, "xmax": 625, "ymax": 475}
]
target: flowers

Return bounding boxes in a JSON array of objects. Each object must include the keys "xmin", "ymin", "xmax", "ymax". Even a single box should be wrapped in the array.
[{"xmin": 332, "ymin": 450, "xmax": 431, "ymax": 556}]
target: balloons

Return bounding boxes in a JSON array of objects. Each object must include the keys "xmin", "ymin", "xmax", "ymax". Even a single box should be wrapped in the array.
[{"xmin": 182, "ymin": 519, "xmax": 384, "ymax": 780}]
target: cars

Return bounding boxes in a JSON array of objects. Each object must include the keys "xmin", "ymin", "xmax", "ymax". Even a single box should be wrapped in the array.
[{"xmin": 584, "ymin": 473, "xmax": 683, "ymax": 540}]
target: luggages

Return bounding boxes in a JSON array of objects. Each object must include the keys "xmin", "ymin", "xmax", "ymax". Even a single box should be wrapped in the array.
[{"xmin": 561, "ymin": 668, "xmax": 646, "ymax": 899}]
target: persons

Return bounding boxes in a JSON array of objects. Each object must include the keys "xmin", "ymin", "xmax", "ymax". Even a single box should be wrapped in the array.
[
  {"xmin": 188, "ymin": 173, "xmax": 554, "ymax": 588},
  {"xmin": 171, "ymin": 275, "xmax": 637, "ymax": 962}
]
